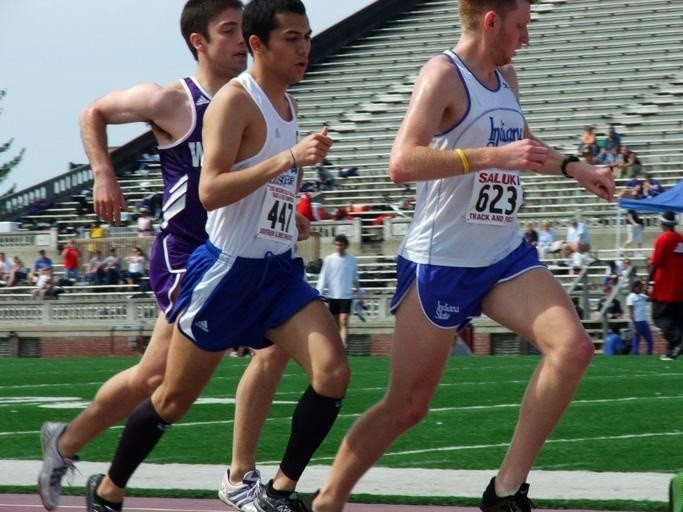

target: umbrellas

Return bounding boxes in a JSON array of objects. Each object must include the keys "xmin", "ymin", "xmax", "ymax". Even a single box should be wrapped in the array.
[{"xmin": 616, "ymin": 179, "xmax": 683, "ymax": 249}]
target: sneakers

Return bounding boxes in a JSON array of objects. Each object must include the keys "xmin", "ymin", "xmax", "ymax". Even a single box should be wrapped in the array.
[
  {"xmin": 254, "ymin": 478, "xmax": 309, "ymax": 512},
  {"xmin": 218, "ymin": 468, "xmax": 263, "ymax": 512},
  {"xmin": 305, "ymin": 488, "xmax": 320, "ymax": 511},
  {"xmin": 36, "ymin": 420, "xmax": 79, "ymax": 511},
  {"xmin": 479, "ymin": 477, "xmax": 534, "ymax": 511},
  {"xmin": 85, "ymin": 473, "xmax": 122, "ymax": 512}
]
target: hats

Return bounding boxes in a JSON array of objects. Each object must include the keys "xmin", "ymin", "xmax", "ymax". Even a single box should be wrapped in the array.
[{"xmin": 656, "ymin": 208, "xmax": 678, "ymax": 226}]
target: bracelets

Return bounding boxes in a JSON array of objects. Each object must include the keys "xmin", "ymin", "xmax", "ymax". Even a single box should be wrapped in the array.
[
  {"xmin": 455, "ymin": 148, "xmax": 470, "ymax": 175},
  {"xmin": 289, "ymin": 148, "xmax": 297, "ymax": 169}
]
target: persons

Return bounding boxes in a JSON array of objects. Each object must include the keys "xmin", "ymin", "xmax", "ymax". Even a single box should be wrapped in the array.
[
  {"xmin": 617, "ymin": 208, "xmax": 644, "ymax": 257},
  {"xmin": 37, "ymin": 0, "xmax": 290, "ymax": 512},
  {"xmin": 274, "ymin": 0, "xmax": 616, "ymax": 512},
  {"xmin": 599, "ymin": 259, "xmax": 654, "ymax": 355},
  {"xmin": 523, "ymin": 218, "xmax": 591, "ymax": 258},
  {"xmin": 645, "ymin": 211, "xmax": 683, "ymax": 360},
  {"xmin": 0, "ymin": 238, "xmax": 146, "ymax": 295},
  {"xmin": 315, "ymin": 233, "xmax": 362, "ymax": 353},
  {"xmin": 86, "ymin": 0, "xmax": 352, "ymax": 512},
  {"xmin": 577, "ymin": 125, "xmax": 663, "ymax": 197}
]
